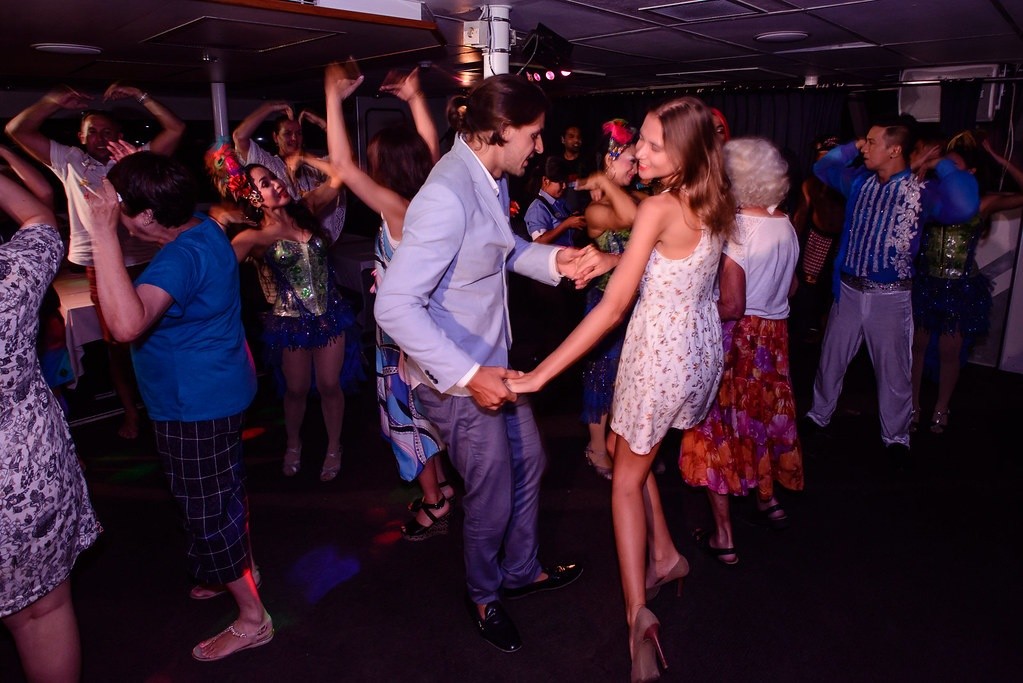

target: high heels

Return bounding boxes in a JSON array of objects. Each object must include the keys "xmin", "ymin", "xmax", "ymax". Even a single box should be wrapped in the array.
[
  {"xmin": 409, "ymin": 481, "xmax": 456, "ymax": 513},
  {"xmin": 618, "ymin": 554, "xmax": 690, "ymax": 605},
  {"xmin": 628, "ymin": 605, "xmax": 670, "ymax": 683},
  {"xmin": 400, "ymin": 494, "xmax": 451, "ymax": 544}
]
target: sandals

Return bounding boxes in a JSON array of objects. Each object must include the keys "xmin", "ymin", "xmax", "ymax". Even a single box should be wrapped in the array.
[
  {"xmin": 191, "ymin": 614, "xmax": 274, "ymax": 662},
  {"xmin": 927, "ymin": 407, "xmax": 951, "ymax": 438},
  {"xmin": 909, "ymin": 407, "xmax": 923, "ymax": 435},
  {"xmin": 690, "ymin": 527, "xmax": 745, "ymax": 572},
  {"xmin": 189, "ymin": 568, "xmax": 263, "ymax": 600},
  {"xmin": 739, "ymin": 502, "xmax": 788, "ymax": 529}
]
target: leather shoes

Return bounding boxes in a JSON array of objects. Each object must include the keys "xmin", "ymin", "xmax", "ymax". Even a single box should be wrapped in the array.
[
  {"xmin": 474, "ymin": 599, "xmax": 523, "ymax": 653},
  {"xmin": 499, "ymin": 563, "xmax": 582, "ymax": 603}
]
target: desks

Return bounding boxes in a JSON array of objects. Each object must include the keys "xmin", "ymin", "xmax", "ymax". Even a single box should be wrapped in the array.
[{"xmin": 51, "ymin": 268, "xmax": 114, "ymax": 419}]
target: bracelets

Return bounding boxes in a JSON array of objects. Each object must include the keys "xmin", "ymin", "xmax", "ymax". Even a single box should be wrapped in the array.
[{"xmin": 137, "ymin": 91, "xmax": 147, "ymax": 101}]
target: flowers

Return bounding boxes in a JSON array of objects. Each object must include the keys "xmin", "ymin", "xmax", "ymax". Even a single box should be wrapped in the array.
[
  {"xmin": 601, "ymin": 116, "xmax": 634, "ymax": 145},
  {"xmin": 510, "ymin": 200, "xmax": 521, "ymax": 219}
]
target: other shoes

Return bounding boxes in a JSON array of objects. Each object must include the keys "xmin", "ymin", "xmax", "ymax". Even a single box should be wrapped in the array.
[
  {"xmin": 583, "ymin": 440, "xmax": 614, "ymax": 481},
  {"xmin": 282, "ymin": 439, "xmax": 302, "ymax": 478},
  {"xmin": 319, "ymin": 443, "xmax": 343, "ymax": 483}
]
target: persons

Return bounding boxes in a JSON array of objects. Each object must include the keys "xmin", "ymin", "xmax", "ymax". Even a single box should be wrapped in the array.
[
  {"xmin": 0, "ymin": 171, "xmax": 105, "ymax": 683},
  {"xmin": 66, "ymin": 150, "xmax": 275, "ymax": 661},
  {"xmin": 0, "ymin": 62, "xmax": 1023, "ymax": 543},
  {"xmin": 803, "ymin": 115, "xmax": 980, "ymax": 459},
  {"xmin": 679, "ymin": 138, "xmax": 800, "ymax": 569},
  {"xmin": 503, "ymin": 92, "xmax": 724, "ymax": 683},
  {"xmin": 374, "ymin": 73, "xmax": 592, "ymax": 652}
]
